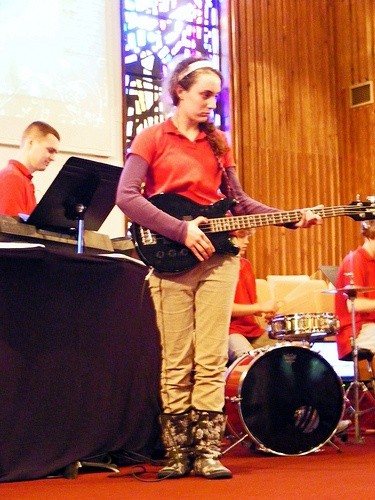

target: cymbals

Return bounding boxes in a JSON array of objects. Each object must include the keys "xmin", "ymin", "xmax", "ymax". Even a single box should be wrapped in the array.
[{"xmin": 322, "ymin": 285, "xmax": 374, "ymax": 294}]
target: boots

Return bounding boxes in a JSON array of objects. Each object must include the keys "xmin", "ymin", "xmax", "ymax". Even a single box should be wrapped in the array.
[
  {"xmin": 190, "ymin": 408, "xmax": 232, "ymax": 479},
  {"xmin": 156, "ymin": 407, "xmax": 194, "ymax": 478}
]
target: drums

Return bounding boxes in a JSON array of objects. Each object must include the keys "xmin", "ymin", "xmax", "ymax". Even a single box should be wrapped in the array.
[
  {"xmin": 225, "ymin": 341, "xmax": 346, "ymax": 457},
  {"xmin": 268, "ymin": 311, "xmax": 340, "ymax": 340}
]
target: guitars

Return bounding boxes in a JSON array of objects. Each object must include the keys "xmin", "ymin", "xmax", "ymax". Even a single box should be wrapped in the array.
[{"xmin": 130, "ymin": 194, "xmax": 375, "ymax": 274}]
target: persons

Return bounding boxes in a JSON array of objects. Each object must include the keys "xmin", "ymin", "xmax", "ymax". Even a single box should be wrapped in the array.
[
  {"xmin": 115, "ymin": 56, "xmax": 322, "ymax": 481},
  {"xmin": 333, "ymin": 218, "xmax": 375, "ymax": 363},
  {"xmin": 1, "ymin": 120, "xmax": 60, "ymax": 217},
  {"xmin": 225, "ymin": 225, "xmax": 279, "ymax": 368}
]
